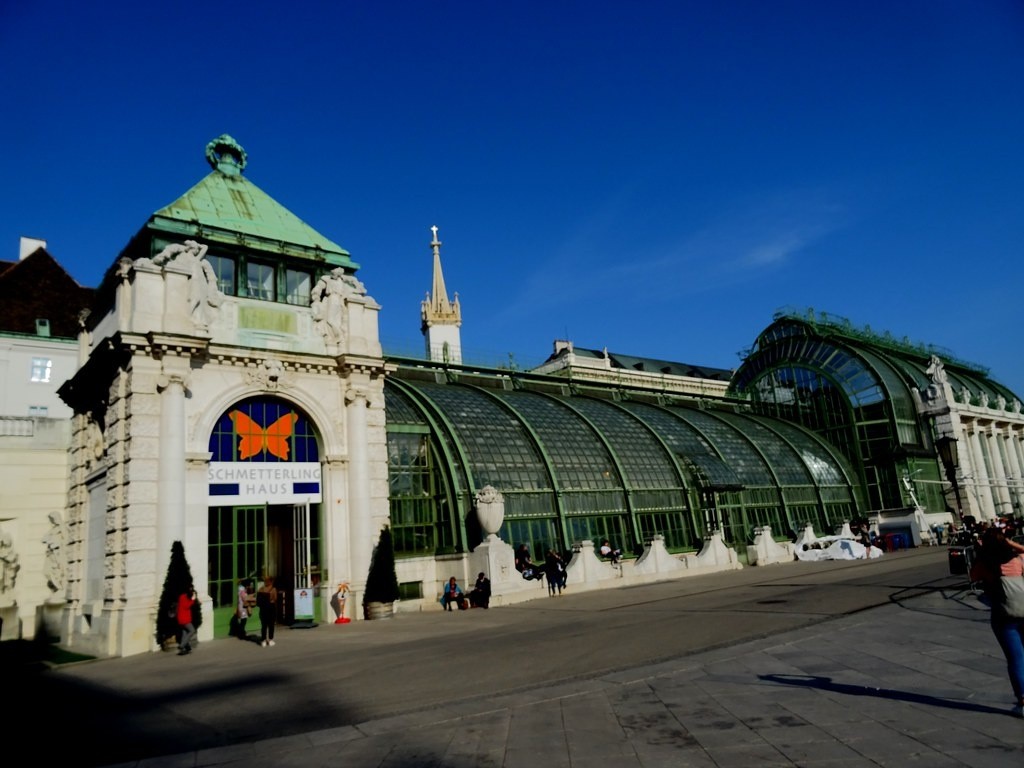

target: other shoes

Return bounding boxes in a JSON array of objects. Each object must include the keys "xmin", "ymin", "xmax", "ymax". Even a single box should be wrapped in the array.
[
  {"xmin": 261, "ymin": 640, "xmax": 267, "ymax": 646},
  {"xmin": 177, "ymin": 646, "xmax": 187, "ymax": 652},
  {"xmin": 1010, "ymin": 703, "xmax": 1024, "ymax": 716},
  {"xmin": 268, "ymin": 639, "xmax": 275, "ymax": 646}
]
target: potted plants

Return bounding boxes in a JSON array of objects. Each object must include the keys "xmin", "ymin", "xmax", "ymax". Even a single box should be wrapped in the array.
[
  {"xmin": 362, "ymin": 525, "xmax": 402, "ymax": 622},
  {"xmin": 157, "ymin": 543, "xmax": 202, "ymax": 651}
]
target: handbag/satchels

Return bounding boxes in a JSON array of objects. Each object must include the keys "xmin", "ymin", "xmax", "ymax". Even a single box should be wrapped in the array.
[
  {"xmin": 247, "ymin": 601, "xmax": 253, "ymax": 616},
  {"xmin": 522, "ymin": 568, "xmax": 534, "ymax": 579}
]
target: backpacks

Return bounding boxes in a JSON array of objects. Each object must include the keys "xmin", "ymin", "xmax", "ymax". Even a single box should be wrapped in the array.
[
  {"xmin": 996, "ymin": 576, "xmax": 1024, "ymax": 619},
  {"xmin": 256, "ymin": 591, "xmax": 270, "ymax": 608},
  {"xmin": 168, "ymin": 599, "xmax": 177, "ymax": 618}
]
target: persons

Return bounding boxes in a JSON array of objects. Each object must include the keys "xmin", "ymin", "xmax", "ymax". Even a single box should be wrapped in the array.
[
  {"xmin": 183, "ymin": 240, "xmax": 211, "ymax": 327},
  {"xmin": 928, "ymin": 513, "xmax": 1024, "ymax": 719},
  {"xmin": 177, "ymin": 585, "xmax": 196, "ymax": 654},
  {"xmin": 860, "ymin": 525, "xmax": 871, "ymax": 559},
  {"xmin": 441, "ymin": 577, "xmax": 465, "ymax": 611},
  {"xmin": 311, "ymin": 266, "xmax": 359, "ymax": 353},
  {"xmin": 473, "ymin": 572, "xmax": 491, "ymax": 610},
  {"xmin": 514, "ymin": 544, "xmax": 567, "ymax": 597},
  {"xmin": 257, "ymin": 576, "xmax": 277, "ymax": 648},
  {"xmin": 237, "ymin": 581, "xmax": 253, "ymax": 638},
  {"xmin": 600, "ymin": 539, "xmax": 622, "ymax": 565}
]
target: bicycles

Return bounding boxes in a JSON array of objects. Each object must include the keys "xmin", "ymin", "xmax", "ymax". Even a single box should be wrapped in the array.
[{"xmin": 947, "ymin": 543, "xmax": 981, "ymax": 590}]
